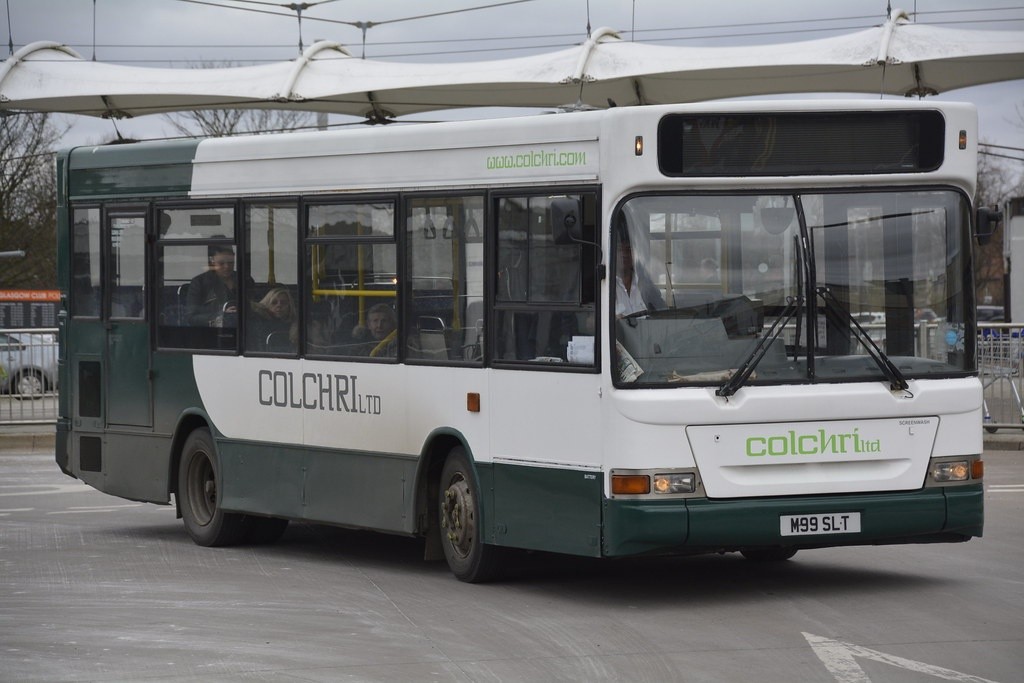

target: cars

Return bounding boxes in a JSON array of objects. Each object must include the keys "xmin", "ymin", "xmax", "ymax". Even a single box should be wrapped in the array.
[{"xmin": 0, "ymin": 332, "xmax": 60, "ymax": 402}]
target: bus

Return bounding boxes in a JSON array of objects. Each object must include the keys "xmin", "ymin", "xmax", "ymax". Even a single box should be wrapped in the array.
[{"xmin": 51, "ymin": 99, "xmax": 1002, "ymax": 586}]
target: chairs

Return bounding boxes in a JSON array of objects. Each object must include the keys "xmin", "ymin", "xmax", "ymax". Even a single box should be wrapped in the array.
[{"xmin": 141, "ymin": 282, "xmax": 450, "ymax": 363}]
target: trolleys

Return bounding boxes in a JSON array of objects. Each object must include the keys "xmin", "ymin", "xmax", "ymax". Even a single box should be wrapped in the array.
[{"xmin": 972, "ymin": 321, "xmax": 1024, "ymax": 435}]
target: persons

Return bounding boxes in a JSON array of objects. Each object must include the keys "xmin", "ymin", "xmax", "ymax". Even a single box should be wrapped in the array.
[
  {"xmin": 701, "ymin": 258, "xmax": 723, "ymax": 285},
  {"xmin": 587, "ymin": 233, "xmax": 670, "ymax": 334},
  {"xmin": 189, "ymin": 236, "xmax": 415, "ymax": 359}
]
